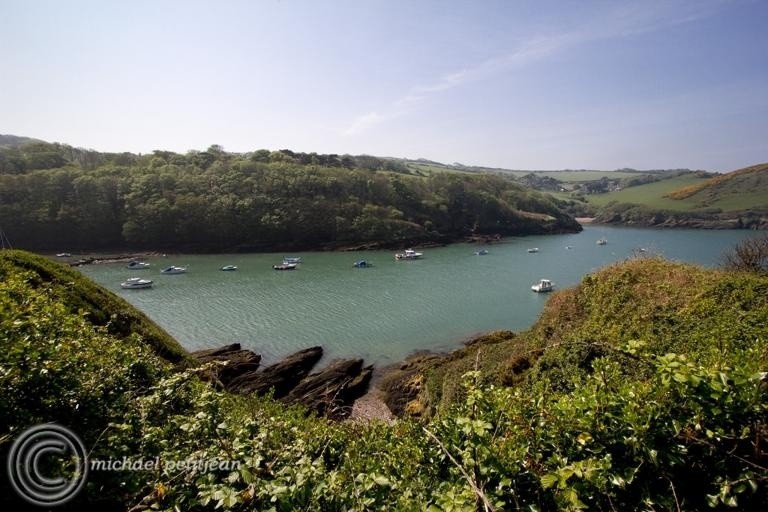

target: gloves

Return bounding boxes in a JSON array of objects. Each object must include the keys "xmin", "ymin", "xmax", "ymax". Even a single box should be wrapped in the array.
[
  {"xmin": 465, "ymin": 236, "xmax": 608, "ymax": 257},
  {"xmin": 270, "ymin": 262, "xmax": 296, "ymax": 270},
  {"xmin": 393, "ymin": 249, "xmax": 423, "ymax": 260},
  {"xmin": 127, "ymin": 260, "xmax": 150, "ymax": 270},
  {"xmin": 528, "ymin": 278, "xmax": 555, "ymax": 293},
  {"xmin": 281, "ymin": 254, "xmax": 303, "ymax": 264},
  {"xmin": 120, "ymin": 276, "xmax": 152, "ymax": 290},
  {"xmin": 351, "ymin": 260, "xmax": 375, "ymax": 268},
  {"xmin": 160, "ymin": 265, "xmax": 186, "ymax": 274},
  {"xmin": 56, "ymin": 252, "xmax": 73, "ymax": 257},
  {"xmin": 220, "ymin": 264, "xmax": 237, "ymax": 273}
]
